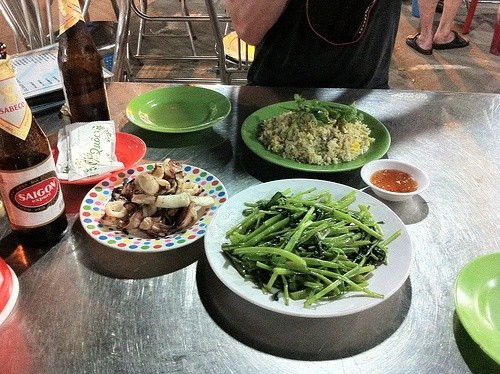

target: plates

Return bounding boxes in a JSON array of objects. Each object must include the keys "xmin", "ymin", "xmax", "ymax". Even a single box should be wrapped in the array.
[
  {"xmin": 203, "ymin": 179, "xmax": 414, "ymax": 318},
  {"xmin": 241, "ymin": 100, "xmax": 392, "ymax": 172},
  {"xmin": 79, "ymin": 162, "xmax": 228, "ymax": 252},
  {"xmin": 360, "ymin": 159, "xmax": 430, "ymax": 201},
  {"xmin": 125, "ymin": 85, "xmax": 231, "ymax": 133},
  {"xmin": 453, "ymin": 251, "xmax": 500, "ymax": 367},
  {"xmin": 50, "ymin": 132, "xmax": 147, "ymax": 184}
]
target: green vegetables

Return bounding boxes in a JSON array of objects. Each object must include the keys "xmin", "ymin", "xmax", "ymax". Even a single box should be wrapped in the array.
[
  {"xmin": 221, "ymin": 185, "xmax": 402, "ymax": 307},
  {"xmin": 278, "ymin": 93, "xmax": 365, "ymax": 128}
]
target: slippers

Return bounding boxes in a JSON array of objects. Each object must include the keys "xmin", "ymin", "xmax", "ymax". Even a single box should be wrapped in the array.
[
  {"xmin": 432, "ymin": 30, "xmax": 469, "ymax": 49},
  {"xmin": 406, "ymin": 32, "xmax": 433, "ymax": 55}
]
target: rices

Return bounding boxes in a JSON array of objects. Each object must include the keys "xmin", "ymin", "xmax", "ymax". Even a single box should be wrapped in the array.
[{"xmin": 258, "ymin": 111, "xmax": 375, "ymax": 166}]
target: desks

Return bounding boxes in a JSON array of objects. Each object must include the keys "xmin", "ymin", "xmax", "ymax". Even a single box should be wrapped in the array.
[{"xmin": 0, "ymin": 82, "xmax": 500, "ymax": 374}]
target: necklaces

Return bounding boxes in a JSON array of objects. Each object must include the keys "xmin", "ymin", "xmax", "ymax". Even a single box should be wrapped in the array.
[{"xmin": 306, "ymin": 0, "xmax": 377, "ymax": 46}]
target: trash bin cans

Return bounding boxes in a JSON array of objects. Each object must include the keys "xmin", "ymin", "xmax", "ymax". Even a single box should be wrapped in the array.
[
  {"xmin": 411, "ymin": 0, "xmax": 420, "ymax": 16},
  {"xmin": 53, "ymin": 21, "xmax": 119, "ymax": 82}
]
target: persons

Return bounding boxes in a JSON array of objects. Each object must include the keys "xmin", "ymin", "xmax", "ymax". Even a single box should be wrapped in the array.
[
  {"xmin": 405, "ymin": 0, "xmax": 469, "ymax": 55},
  {"xmin": 225, "ymin": 0, "xmax": 402, "ymax": 90}
]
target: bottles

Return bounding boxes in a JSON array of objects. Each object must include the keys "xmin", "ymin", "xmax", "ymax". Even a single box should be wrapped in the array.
[
  {"xmin": 0, "ymin": 42, "xmax": 69, "ymax": 247},
  {"xmin": 57, "ymin": 0, "xmax": 110, "ymax": 124}
]
